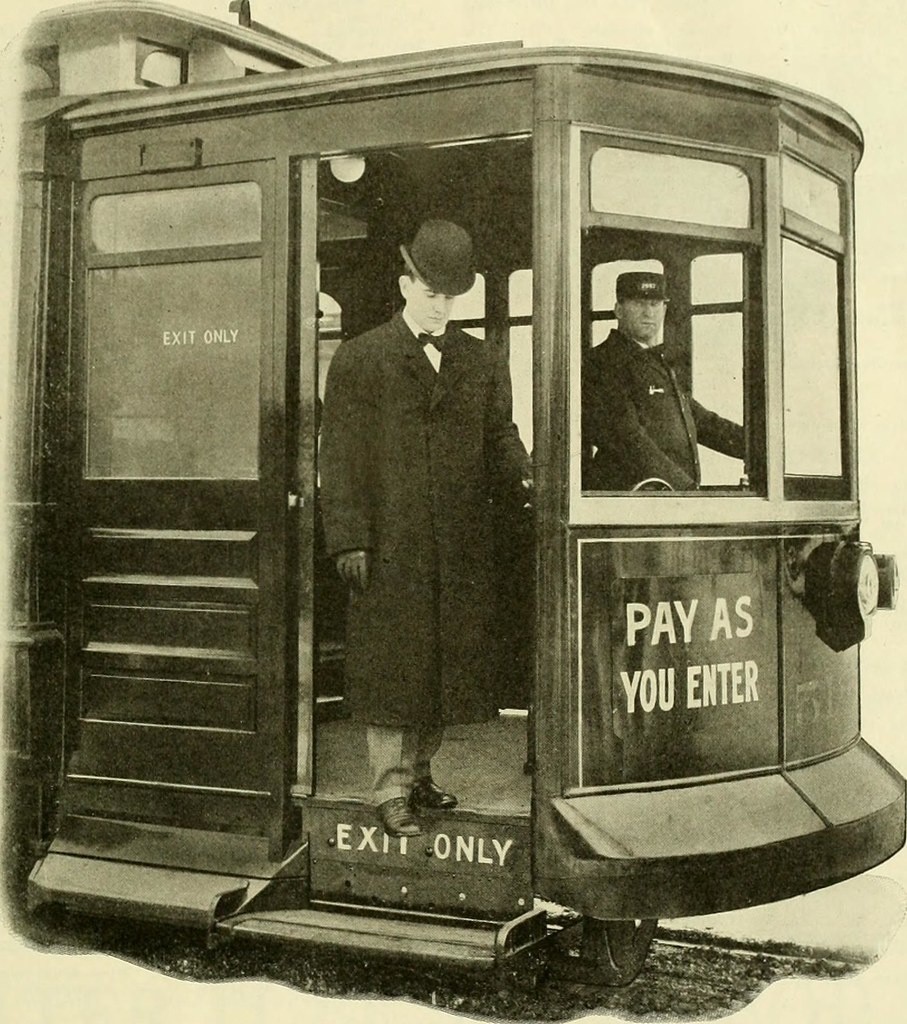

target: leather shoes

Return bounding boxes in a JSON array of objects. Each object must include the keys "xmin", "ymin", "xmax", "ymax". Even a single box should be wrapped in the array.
[
  {"xmin": 413, "ymin": 780, "xmax": 459, "ymax": 808},
  {"xmin": 377, "ymin": 796, "xmax": 422, "ymax": 837}
]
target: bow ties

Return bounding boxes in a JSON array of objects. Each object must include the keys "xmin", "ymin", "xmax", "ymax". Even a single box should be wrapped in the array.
[{"xmin": 418, "ymin": 333, "xmax": 447, "ymax": 351}]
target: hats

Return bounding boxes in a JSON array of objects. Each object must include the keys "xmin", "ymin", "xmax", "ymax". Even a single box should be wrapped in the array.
[
  {"xmin": 615, "ymin": 272, "xmax": 670, "ymax": 303},
  {"xmin": 398, "ymin": 218, "xmax": 475, "ymax": 296}
]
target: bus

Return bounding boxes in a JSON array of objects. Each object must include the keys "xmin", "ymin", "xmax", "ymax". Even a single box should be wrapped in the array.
[{"xmin": 0, "ymin": 0, "xmax": 907, "ymax": 997}]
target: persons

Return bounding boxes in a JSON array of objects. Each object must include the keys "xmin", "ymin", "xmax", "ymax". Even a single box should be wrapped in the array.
[
  {"xmin": 317, "ymin": 217, "xmax": 532, "ymax": 834},
  {"xmin": 581, "ymin": 272, "xmax": 745, "ymax": 491}
]
